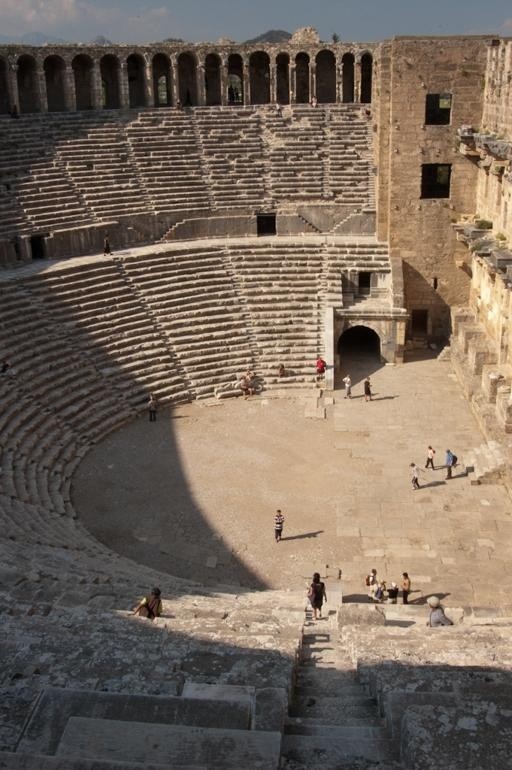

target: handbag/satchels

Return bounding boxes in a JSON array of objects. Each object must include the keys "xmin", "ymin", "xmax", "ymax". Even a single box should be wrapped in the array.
[
  {"xmin": 452, "ymin": 456, "xmax": 457, "ymax": 465},
  {"xmin": 140, "ymin": 606, "xmax": 150, "ymax": 618}
]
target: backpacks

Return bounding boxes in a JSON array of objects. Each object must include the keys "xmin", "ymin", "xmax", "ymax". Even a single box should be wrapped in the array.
[
  {"xmin": 375, "ymin": 586, "xmax": 384, "ymax": 599},
  {"xmin": 365, "ymin": 575, "xmax": 374, "ymax": 586}
]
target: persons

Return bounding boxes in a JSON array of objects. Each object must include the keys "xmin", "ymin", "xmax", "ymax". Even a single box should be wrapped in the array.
[
  {"xmin": 147, "ymin": 396, "xmax": 157, "ymax": 422},
  {"xmin": 133, "ymin": 587, "xmax": 163, "ymax": 618},
  {"xmin": 426, "ymin": 595, "xmax": 454, "ymax": 627},
  {"xmin": 307, "ymin": 573, "xmax": 327, "ymax": 620},
  {"xmin": 410, "ymin": 446, "xmax": 453, "ymax": 490},
  {"xmin": 273, "ymin": 509, "xmax": 284, "ymax": 543},
  {"xmin": 366, "ymin": 568, "xmax": 411, "ymax": 604},
  {"xmin": 343, "ymin": 374, "xmax": 352, "ymax": 399},
  {"xmin": 103, "ymin": 234, "xmax": 113, "ymax": 256},
  {"xmin": 279, "ymin": 364, "xmax": 286, "ymax": 378},
  {"xmin": 364, "ymin": 376, "xmax": 372, "ymax": 402},
  {"xmin": 229, "ymin": 85, "xmax": 240, "ymax": 102},
  {"xmin": 315, "ymin": 358, "xmax": 326, "ymax": 380},
  {"xmin": 240, "ymin": 369, "xmax": 257, "ymax": 400}
]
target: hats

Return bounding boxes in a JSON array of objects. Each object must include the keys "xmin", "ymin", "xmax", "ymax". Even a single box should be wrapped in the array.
[
  {"xmin": 426, "ymin": 596, "xmax": 440, "ymax": 608},
  {"xmin": 391, "ymin": 582, "xmax": 397, "ymax": 588}
]
